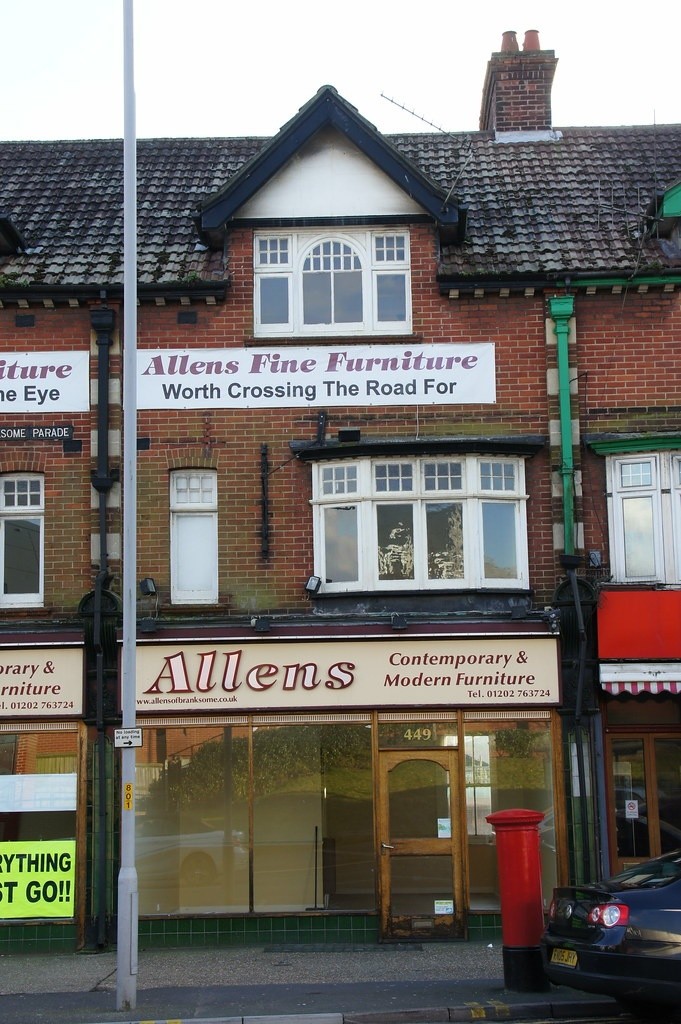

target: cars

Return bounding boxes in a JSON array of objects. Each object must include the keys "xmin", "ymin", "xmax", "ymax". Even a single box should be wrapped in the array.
[
  {"xmin": 537, "ymin": 786, "xmax": 681, "ymax": 881},
  {"xmin": 133, "ymin": 814, "xmax": 249, "ymax": 900},
  {"xmin": 544, "ymin": 848, "xmax": 681, "ymax": 1014}
]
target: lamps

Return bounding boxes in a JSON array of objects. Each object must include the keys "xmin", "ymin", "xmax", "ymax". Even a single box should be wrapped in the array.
[
  {"xmin": 511, "ymin": 606, "xmax": 527, "ymax": 619},
  {"xmin": 305, "ymin": 577, "xmax": 320, "ymax": 601},
  {"xmin": 140, "ymin": 578, "xmax": 158, "ymax": 632},
  {"xmin": 251, "ymin": 615, "xmax": 271, "ymax": 633},
  {"xmin": 390, "ymin": 612, "xmax": 408, "ymax": 629}
]
